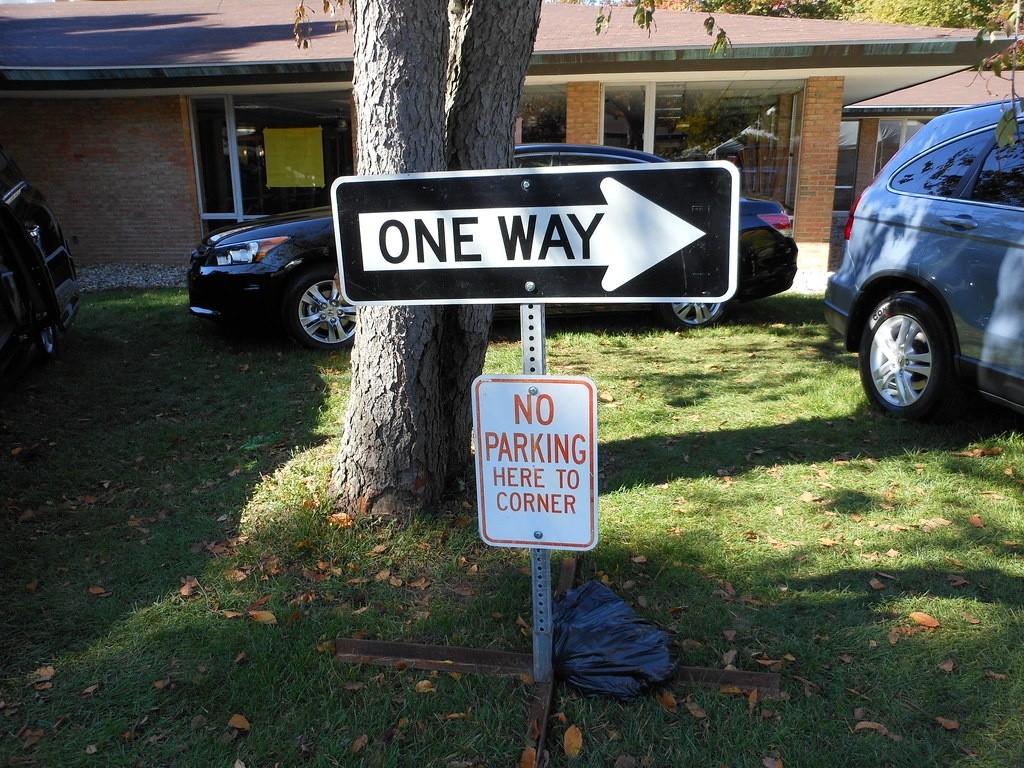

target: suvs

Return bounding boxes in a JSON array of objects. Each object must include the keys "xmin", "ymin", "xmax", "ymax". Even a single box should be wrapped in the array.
[{"xmin": 823, "ymin": 96, "xmax": 1024, "ymax": 427}]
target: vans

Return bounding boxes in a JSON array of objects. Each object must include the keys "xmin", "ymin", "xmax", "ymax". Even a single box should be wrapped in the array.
[{"xmin": 0, "ymin": 150, "xmax": 82, "ymax": 385}]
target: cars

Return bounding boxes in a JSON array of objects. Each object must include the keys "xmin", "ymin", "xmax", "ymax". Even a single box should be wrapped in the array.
[{"xmin": 187, "ymin": 143, "xmax": 798, "ymax": 352}]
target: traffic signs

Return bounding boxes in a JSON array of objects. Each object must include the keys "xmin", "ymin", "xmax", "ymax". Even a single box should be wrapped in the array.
[{"xmin": 330, "ymin": 159, "xmax": 741, "ymax": 306}]
